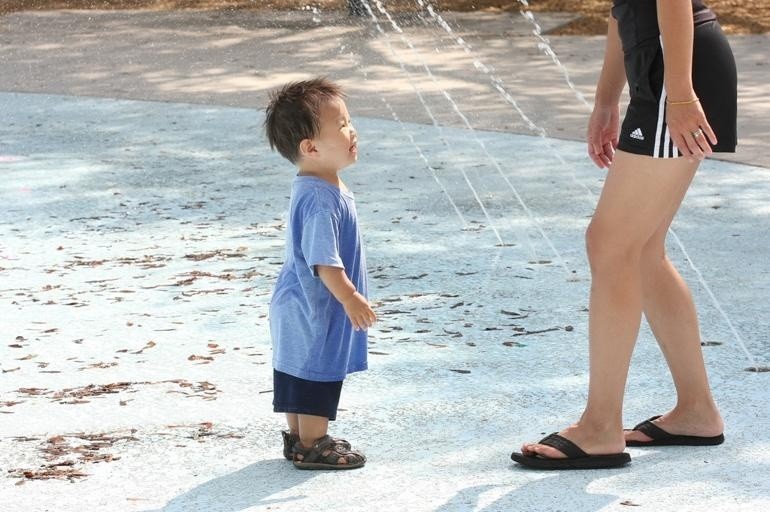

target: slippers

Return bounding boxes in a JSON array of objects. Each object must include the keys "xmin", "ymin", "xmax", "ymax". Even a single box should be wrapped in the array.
[
  {"xmin": 511, "ymin": 431, "xmax": 631, "ymax": 467},
  {"xmin": 627, "ymin": 415, "xmax": 725, "ymax": 446}
]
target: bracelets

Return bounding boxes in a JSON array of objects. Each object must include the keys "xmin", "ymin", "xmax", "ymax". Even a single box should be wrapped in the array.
[{"xmin": 666, "ymin": 98, "xmax": 700, "ymax": 105}]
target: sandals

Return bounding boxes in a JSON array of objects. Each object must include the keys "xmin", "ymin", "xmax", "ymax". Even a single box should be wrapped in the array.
[{"xmin": 279, "ymin": 432, "xmax": 367, "ymax": 470}]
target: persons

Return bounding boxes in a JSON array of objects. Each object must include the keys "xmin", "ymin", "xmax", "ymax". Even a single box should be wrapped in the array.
[
  {"xmin": 508, "ymin": 0, "xmax": 740, "ymax": 469},
  {"xmin": 261, "ymin": 75, "xmax": 378, "ymax": 470}
]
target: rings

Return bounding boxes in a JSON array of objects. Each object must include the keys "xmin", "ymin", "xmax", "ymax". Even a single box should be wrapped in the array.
[{"xmin": 694, "ymin": 129, "xmax": 703, "ymax": 137}]
